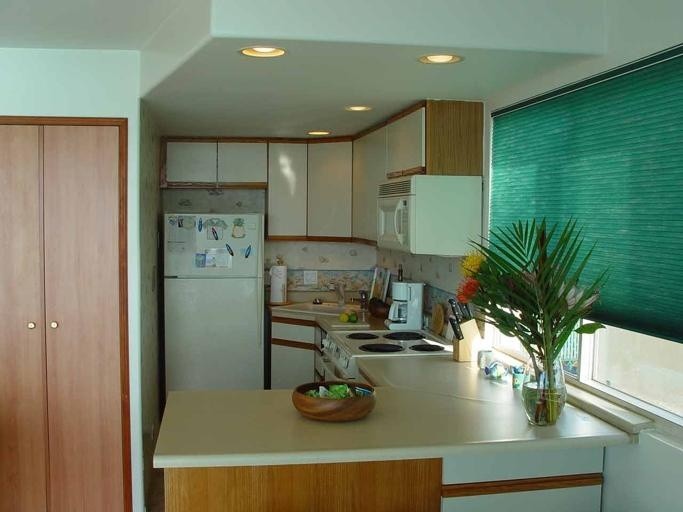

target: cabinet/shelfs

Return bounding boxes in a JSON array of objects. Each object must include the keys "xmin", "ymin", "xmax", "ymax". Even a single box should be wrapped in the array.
[
  {"xmin": 308, "ymin": 136, "xmax": 352, "ymax": 241},
  {"xmin": 352, "ymin": 121, "xmax": 386, "ymax": 246},
  {"xmin": 268, "ymin": 137, "xmax": 307, "ymax": 241},
  {"xmin": 160, "ymin": 137, "xmax": 268, "ymax": 189},
  {"xmin": 388, "ymin": 100, "xmax": 484, "ymax": 175},
  {"xmin": 270, "ymin": 307, "xmax": 315, "ymax": 390},
  {"xmin": 0, "ymin": 115, "xmax": 133, "ymax": 512}
]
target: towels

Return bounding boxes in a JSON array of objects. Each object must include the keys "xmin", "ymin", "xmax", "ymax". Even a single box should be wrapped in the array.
[
  {"xmin": 325, "ymin": 367, "xmax": 345, "ymax": 383},
  {"xmin": 314, "ymin": 351, "xmax": 326, "ymax": 376}
]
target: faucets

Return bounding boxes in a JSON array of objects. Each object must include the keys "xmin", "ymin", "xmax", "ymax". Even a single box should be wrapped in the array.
[
  {"xmin": 351, "ymin": 290, "xmax": 366, "ymax": 309},
  {"xmin": 328, "ymin": 280, "xmax": 346, "ymax": 305}
]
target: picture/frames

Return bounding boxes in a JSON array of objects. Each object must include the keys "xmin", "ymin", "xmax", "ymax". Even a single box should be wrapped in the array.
[{"xmin": 370, "ymin": 268, "xmax": 391, "ymax": 302}]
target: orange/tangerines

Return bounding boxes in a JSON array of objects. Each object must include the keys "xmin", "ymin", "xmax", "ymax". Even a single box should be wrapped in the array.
[{"xmin": 339, "ymin": 311, "xmax": 358, "ymax": 323}]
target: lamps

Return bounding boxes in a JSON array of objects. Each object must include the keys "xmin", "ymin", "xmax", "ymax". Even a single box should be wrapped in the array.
[
  {"xmin": 418, "ymin": 53, "xmax": 464, "ymax": 64},
  {"xmin": 239, "ymin": 45, "xmax": 284, "ymax": 59}
]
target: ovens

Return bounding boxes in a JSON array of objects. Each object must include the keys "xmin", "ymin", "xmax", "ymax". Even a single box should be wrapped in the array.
[{"xmin": 318, "ymin": 348, "xmax": 355, "ymax": 382}]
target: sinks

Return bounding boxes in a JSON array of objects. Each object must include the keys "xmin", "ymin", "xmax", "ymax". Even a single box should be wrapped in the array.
[{"xmin": 281, "ymin": 302, "xmax": 350, "ymax": 316}]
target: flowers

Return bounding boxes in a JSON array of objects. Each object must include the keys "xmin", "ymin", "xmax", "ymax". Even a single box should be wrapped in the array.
[{"xmin": 456, "ymin": 215, "xmax": 612, "ymax": 423}]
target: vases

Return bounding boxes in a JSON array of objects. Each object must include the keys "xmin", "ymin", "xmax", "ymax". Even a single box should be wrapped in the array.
[{"xmin": 521, "ymin": 357, "xmax": 567, "ymax": 426}]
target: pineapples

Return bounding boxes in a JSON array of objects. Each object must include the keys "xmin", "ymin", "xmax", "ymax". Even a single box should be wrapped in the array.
[{"xmin": 232, "ymin": 225, "xmax": 245, "ymax": 238}]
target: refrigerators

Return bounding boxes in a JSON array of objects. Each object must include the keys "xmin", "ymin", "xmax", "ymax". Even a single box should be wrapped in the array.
[{"xmin": 161, "ymin": 214, "xmax": 265, "ymax": 390}]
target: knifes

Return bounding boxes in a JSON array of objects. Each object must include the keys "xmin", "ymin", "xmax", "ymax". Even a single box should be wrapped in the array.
[{"xmin": 447, "ymin": 297, "xmax": 471, "ymax": 341}]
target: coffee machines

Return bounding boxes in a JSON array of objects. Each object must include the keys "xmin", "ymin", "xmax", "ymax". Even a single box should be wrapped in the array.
[{"xmin": 383, "ymin": 281, "xmax": 426, "ymax": 330}]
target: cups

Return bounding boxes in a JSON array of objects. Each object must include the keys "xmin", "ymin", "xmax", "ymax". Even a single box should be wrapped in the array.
[{"xmin": 476, "ymin": 350, "xmax": 530, "ymax": 390}]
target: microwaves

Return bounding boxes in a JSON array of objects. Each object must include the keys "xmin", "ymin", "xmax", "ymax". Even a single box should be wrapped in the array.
[{"xmin": 375, "ymin": 174, "xmax": 483, "ymax": 256}]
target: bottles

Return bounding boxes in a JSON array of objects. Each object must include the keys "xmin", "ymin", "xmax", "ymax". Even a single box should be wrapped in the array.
[{"xmin": 337, "ymin": 280, "xmax": 345, "ymax": 305}]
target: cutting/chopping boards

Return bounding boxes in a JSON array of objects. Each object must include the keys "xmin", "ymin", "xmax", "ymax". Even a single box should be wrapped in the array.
[{"xmin": 323, "ymin": 317, "xmax": 371, "ymax": 328}]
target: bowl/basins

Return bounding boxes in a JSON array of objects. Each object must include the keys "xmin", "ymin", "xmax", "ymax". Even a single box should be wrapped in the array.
[{"xmin": 291, "ymin": 381, "xmax": 376, "ymax": 422}]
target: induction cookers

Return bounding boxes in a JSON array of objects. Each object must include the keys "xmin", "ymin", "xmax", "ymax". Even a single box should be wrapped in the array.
[{"xmin": 323, "ymin": 330, "xmax": 453, "ymax": 371}]
